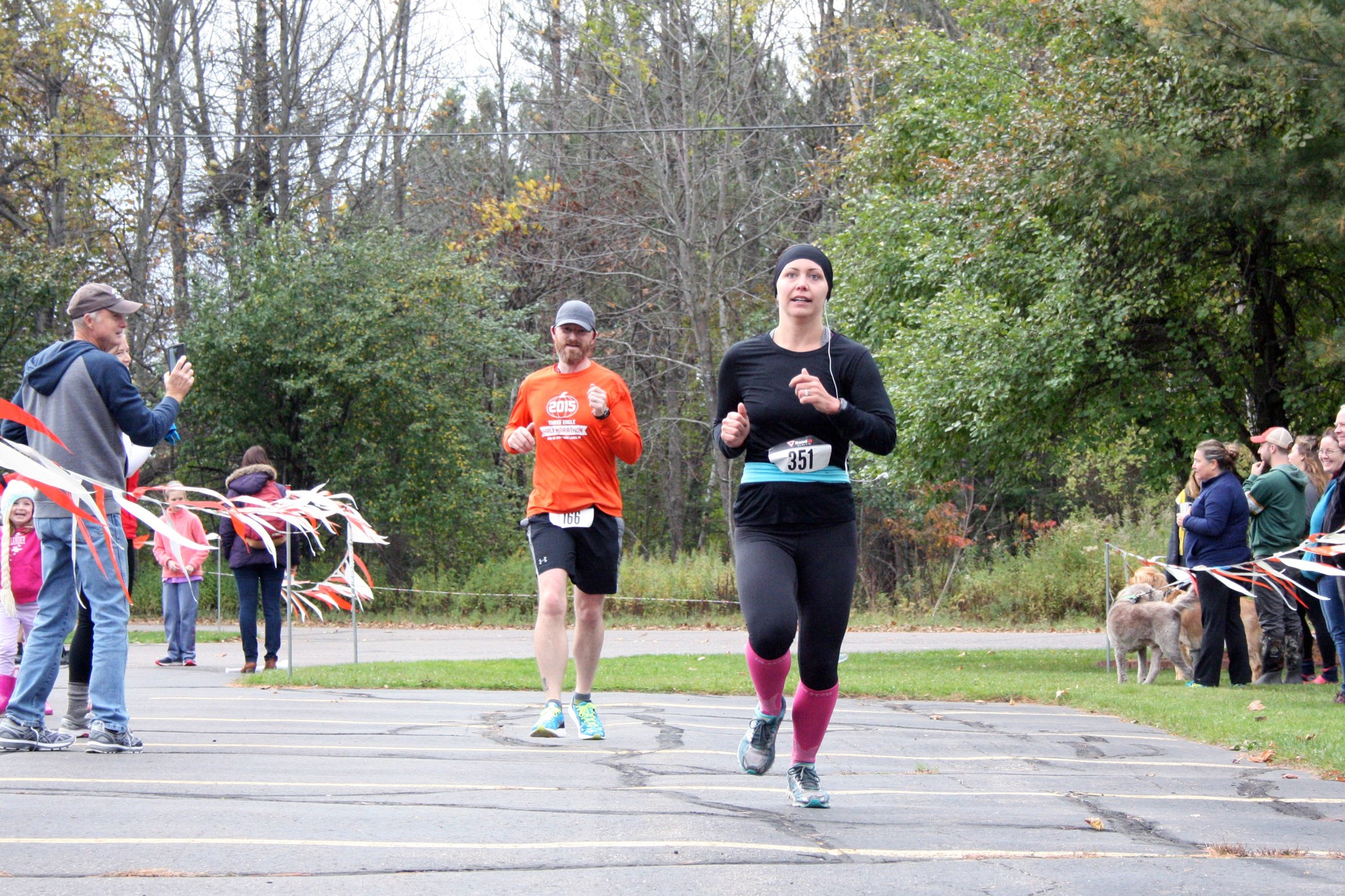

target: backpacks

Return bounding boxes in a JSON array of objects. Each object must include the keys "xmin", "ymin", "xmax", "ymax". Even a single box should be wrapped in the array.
[{"xmin": 242, "ymin": 480, "xmax": 287, "ymax": 548}]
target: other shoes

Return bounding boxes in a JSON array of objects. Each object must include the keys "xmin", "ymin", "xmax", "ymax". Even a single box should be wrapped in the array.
[
  {"xmin": 60, "ymin": 651, "xmax": 69, "ymax": 664},
  {"xmin": 1333, "ymin": 691, "xmax": 1345, "ymax": 703},
  {"xmin": 1253, "ymin": 671, "xmax": 1283, "ymax": 684},
  {"xmin": 15, "ymin": 655, "xmax": 22, "ymax": 664},
  {"xmin": 1284, "ymin": 668, "xmax": 1303, "ymax": 684}
]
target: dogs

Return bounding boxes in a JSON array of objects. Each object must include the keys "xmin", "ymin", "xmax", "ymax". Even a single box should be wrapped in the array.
[
  {"xmin": 1126, "ymin": 566, "xmax": 1264, "ymax": 683},
  {"xmin": 1106, "ymin": 585, "xmax": 1195, "ymax": 686}
]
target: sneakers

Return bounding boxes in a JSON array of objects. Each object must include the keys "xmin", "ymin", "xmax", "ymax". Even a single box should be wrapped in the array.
[
  {"xmin": 737, "ymin": 694, "xmax": 786, "ymax": 774},
  {"xmin": 568, "ymin": 695, "xmax": 606, "ymax": 739},
  {"xmin": 85, "ymin": 725, "xmax": 143, "ymax": 753},
  {"xmin": 1231, "ymin": 683, "xmax": 1246, "ymax": 687},
  {"xmin": 0, "ymin": 718, "xmax": 76, "ymax": 751},
  {"xmin": 530, "ymin": 702, "xmax": 568, "ymax": 737},
  {"xmin": 785, "ymin": 762, "xmax": 831, "ymax": 807},
  {"xmin": 58, "ymin": 714, "xmax": 92, "ymax": 736},
  {"xmin": 1302, "ymin": 673, "xmax": 1339, "ymax": 684},
  {"xmin": 184, "ymin": 657, "xmax": 197, "ymax": 666},
  {"xmin": 155, "ymin": 657, "xmax": 183, "ymax": 666},
  {"xmin": 1185, "ymin": 680, "xmax": 1206, "ymax": 687}
]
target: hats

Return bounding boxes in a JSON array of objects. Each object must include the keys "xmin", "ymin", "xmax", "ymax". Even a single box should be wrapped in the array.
[
  {"xmin": 1, "ymin": 479, "xmax": 34, "ymax": 619},
  {"xmin": 555, "ymin": 299, "xmax": 596, "ymax": 331},
  {"xmin": 68, "ymin": 283, "xmax": 143, "ymax": 319},
  {"xmin": 1250, "ymin": 426, "xmax": 1293, "ymax": 450}
]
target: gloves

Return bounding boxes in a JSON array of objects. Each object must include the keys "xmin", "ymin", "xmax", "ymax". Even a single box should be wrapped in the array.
[{"xmin": 164, "ymin": 423, "xmax": 181, "ymax": 444}]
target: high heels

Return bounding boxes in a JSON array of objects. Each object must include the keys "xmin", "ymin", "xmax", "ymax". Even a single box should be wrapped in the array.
[
  {"xmin": 264, "ymin": 660, "xmax": 276, "ymax": 669},
  {"xmin": 241, "ymin": 661, "xmax": 256, "ymax": 673}
]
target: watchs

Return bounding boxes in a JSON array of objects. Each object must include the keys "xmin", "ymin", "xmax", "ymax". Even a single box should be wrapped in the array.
[
  {"xmin": 838, "ymin": 398, "xmax": 848, "ymax": 411},
  {"xmin": 592, "ymin": 408, "xmax": 610, "ymax": 420}
]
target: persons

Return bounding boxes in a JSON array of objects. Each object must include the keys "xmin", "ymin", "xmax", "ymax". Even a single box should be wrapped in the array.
[
  {"xmin": 1174, "ymin": 402, "xmax": 1345, "ymax": 703},
  {"xmin": 501, "ymin": 299, "xmax": 642, "ymax": 742},
  {"xmin": 0, "ymin": 281, "xmax": 302, "ymax": 754},
  {"xmin": 709, "ymin": 244, "xmax": 896, "ymax": 808}
]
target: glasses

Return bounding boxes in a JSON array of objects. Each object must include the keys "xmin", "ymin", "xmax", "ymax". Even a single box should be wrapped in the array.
[{"xmin": 1316, "ymin": 449, "xmax": 1344, "ymax": 457}]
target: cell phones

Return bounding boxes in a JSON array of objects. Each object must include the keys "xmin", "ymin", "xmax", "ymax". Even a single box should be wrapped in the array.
[
  {"xmin": 1180, "ymin": 503, "xmax": 1188, "ymax": 514},
  {"xmin": 168, "ymin": 342, "xmax": 186, "ymax": 375}
]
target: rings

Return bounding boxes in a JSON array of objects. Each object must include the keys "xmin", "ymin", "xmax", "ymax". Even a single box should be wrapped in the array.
[{"xmin": 805, "ymin": 389, "xmax": 809, "ymax": 396}]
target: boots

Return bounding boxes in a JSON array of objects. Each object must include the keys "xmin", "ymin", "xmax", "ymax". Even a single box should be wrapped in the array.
[
  {"xmin": 0, "ymin": 674, "xmax": 17, "ymax": 717},
  {"xmin": 45, "ymin": 701, "xmax": 53, "ymax": 714}
]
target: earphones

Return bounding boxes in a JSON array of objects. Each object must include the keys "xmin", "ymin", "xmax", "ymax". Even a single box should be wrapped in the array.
[
  {"xmin": 824, "ymin": 299, "xmax": 828, "ymax": 304},
  {"xmin": 875, "ymin": 472, "xmax": 888, "ymax": 480}
]
